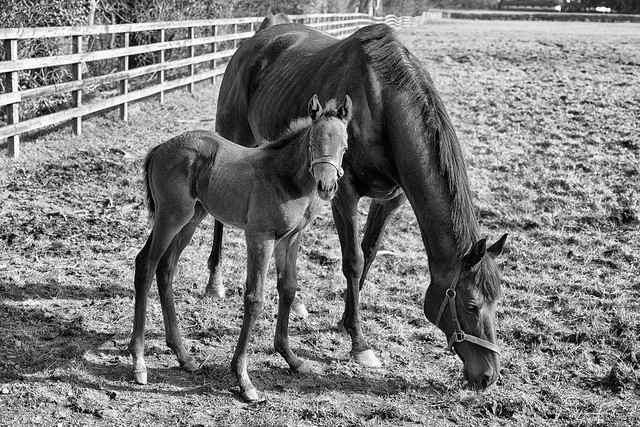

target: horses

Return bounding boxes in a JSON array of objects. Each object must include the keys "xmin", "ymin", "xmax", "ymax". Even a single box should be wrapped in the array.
[
  {"xmin": 205, "ymin": 12, "xmax": 509, "ymax": 391},
  {"xmin": 127, "ymin": 94, "xmax": 354, "ymax": 402}
]
